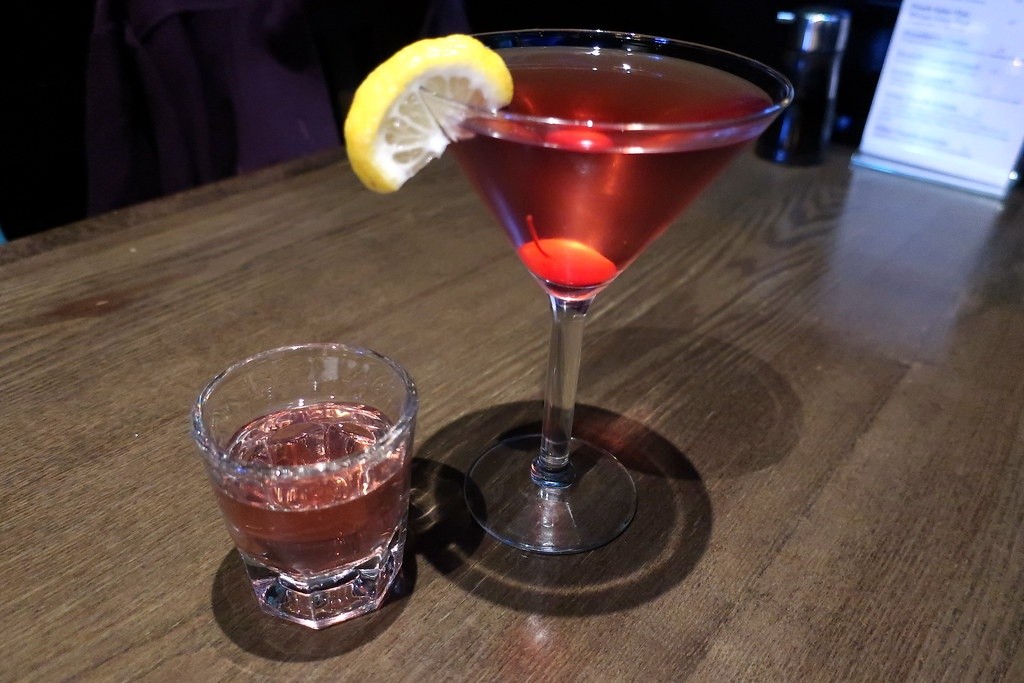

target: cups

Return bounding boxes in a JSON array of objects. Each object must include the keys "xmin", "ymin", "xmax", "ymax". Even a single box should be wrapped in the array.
[
  {"xmin": 192, "ymin": 342, "xmax": 419, "ymax": 630},
  {"xmin": 756, "ymin": 8, "xmax": 851, "ymax": 166}
]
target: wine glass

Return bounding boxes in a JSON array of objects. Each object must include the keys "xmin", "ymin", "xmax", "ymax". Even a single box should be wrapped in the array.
[{"xmin": 455, "ymin": 29, "xmax": 795, "ymax": 556}]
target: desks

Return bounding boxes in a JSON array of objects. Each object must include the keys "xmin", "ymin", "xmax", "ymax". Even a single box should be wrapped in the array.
[{"xmin": 0, "ymin": 139, "xmax": 1024, "ymax": 683}]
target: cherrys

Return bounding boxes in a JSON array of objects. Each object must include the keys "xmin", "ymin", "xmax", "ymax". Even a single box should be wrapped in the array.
[{"xmin": 518, "ymin": 214, "xmax": 615, "ymax": 286}]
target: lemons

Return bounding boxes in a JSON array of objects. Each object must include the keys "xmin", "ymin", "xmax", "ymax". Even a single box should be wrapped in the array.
[{"xmin": 343, "ymin": 35, "xmax": 514, "ymax": 193}]
